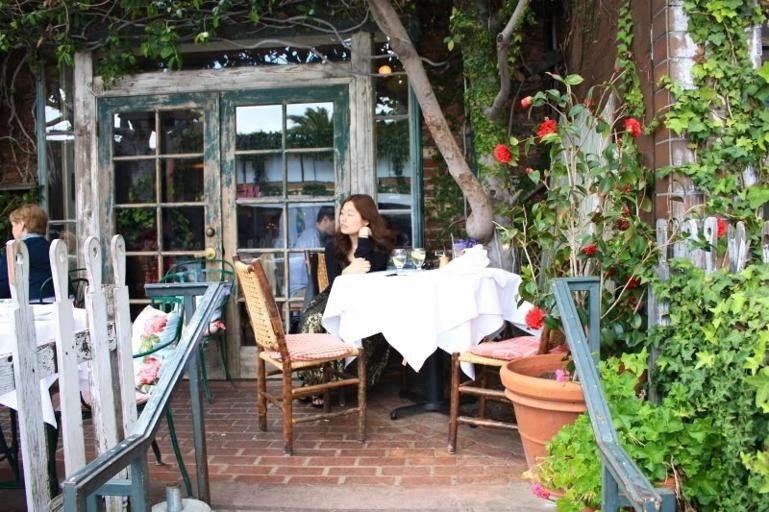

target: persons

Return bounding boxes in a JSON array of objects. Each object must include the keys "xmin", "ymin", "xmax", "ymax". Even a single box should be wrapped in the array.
[
  {"xmin": 288, "ymin": 205, "xmax": 337, "ymax": 319},
  {"xmin": 291, "ymin": 195, "xmax": 402, "ymax": 408},
  {"xmin": 0, "ymin": 204, "xmax": 69, "ymax": 305}
]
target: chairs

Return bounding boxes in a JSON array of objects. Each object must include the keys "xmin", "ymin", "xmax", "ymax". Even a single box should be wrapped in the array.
[
  {"xmin": 151, "ymin": 258, "xmax": 239, "ymax": 405},
  {"xmin": 302, "ymin": 249, "xmax": 409, "ymax": 406},
  {"xmin": 232, "ymin": 255, "xmax": 368, "ymax": 456},
  {"xmin": 38, "ymin": 297, "xmax": 197, "ymax": 502},
  {"xmin": 447, "ymin": 307, "xmax": 566, "ymax": 456},
  {"xmin": 37, "ymin": 267, "xmax": 95, "ymax": 306}
]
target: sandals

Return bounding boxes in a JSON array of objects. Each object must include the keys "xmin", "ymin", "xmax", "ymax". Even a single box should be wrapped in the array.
[{"xmin": 311, "ymin": 395, "xmax": 324, "ymax": 408}]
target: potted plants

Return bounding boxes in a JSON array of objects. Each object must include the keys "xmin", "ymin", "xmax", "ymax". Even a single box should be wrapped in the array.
[{"xmin": 494, "ymin": 67, "xmax": 731, "ymax": 495}]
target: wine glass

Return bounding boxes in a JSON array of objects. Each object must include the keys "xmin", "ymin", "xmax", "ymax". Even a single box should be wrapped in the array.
[
  {"xmin": 391, "ymin": 248, "xmax": 408, "ymax": 277},
  {"xmin": 410, "ymin": 247, "xmax": 427, "ymax": 273}
]
